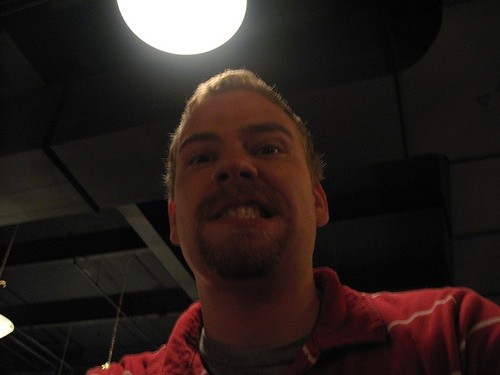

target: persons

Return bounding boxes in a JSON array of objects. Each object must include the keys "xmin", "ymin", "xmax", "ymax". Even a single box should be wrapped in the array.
[{"xmin": 82, "ymin": 67, "xmax": 500, "ymax": 375}]
